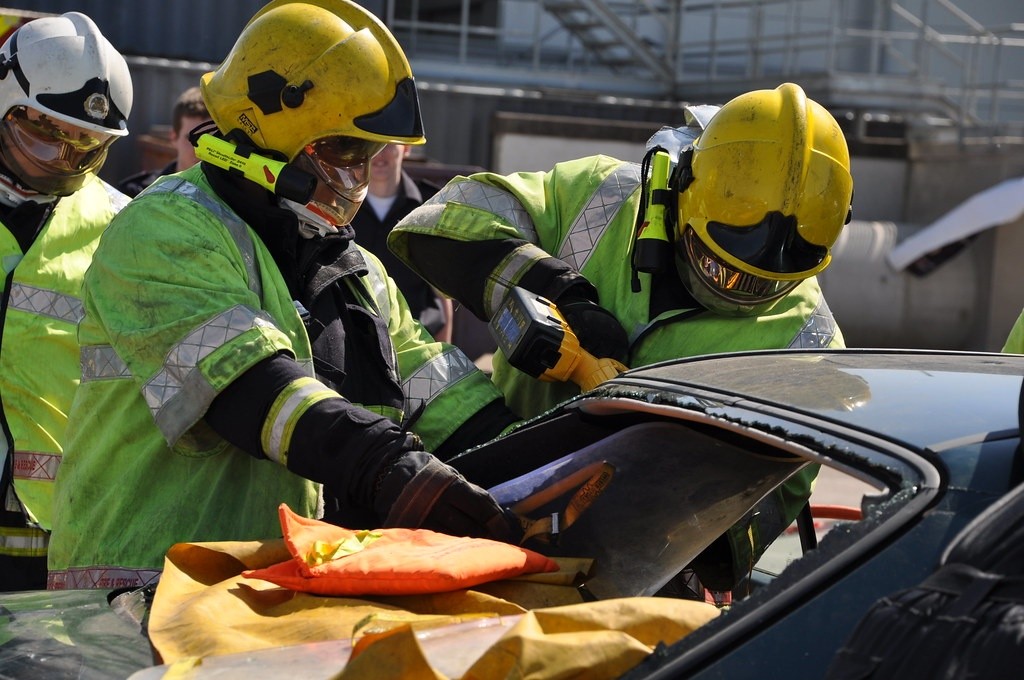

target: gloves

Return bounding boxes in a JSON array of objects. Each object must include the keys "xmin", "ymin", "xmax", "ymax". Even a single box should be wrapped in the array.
[
  {"xmin": 548, "ymin": 271, "xmax": 636, "ymax": 365},
  {"xmin": 321, "ymin": 405, "xmax": 522, "ymax": 542}
]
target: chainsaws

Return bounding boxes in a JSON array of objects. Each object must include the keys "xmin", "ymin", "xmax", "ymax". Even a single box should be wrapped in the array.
[{"xmin": 486, "ymin": 284, "xmax": 631, "ymax": 394}]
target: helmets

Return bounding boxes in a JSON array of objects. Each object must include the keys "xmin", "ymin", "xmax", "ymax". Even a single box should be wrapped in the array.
[
  {"xmin": 198, "ymin": 1, "xmax": 428, "ymax": 163},
  {"xmin": 673, "ymin": 81, "xmax": 854, "ymax": 282},
  {"xmin": 1, "ymin": 10, "xmax": 133, "ymax": 137}
]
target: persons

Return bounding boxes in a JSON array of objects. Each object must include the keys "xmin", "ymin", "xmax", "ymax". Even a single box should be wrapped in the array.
[
  {"xmin": 0, "ymin": 0, "xmax": 524, "ymax": 590},
  {"xmin": 387, "ymin": 83, "xmax": 849, "ymax": 594}
]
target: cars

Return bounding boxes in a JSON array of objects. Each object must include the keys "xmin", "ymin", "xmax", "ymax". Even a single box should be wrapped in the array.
[{"xmin": 0, "ymin": 348, "xmax": 1023, "ymax": 680}]
